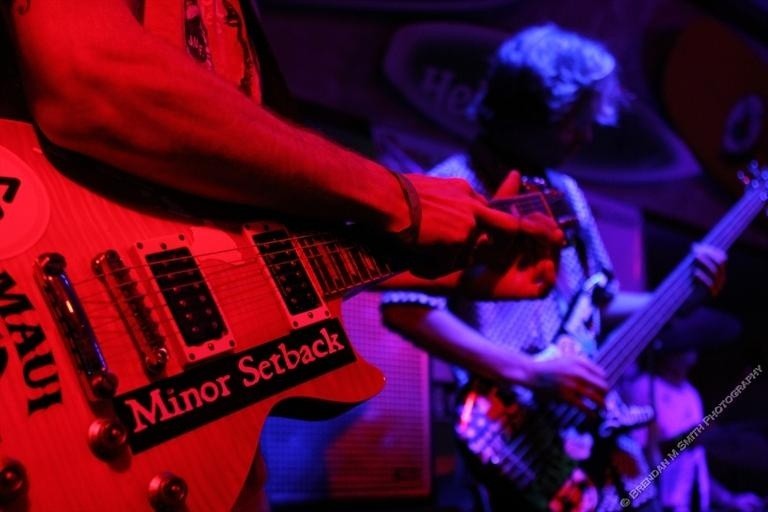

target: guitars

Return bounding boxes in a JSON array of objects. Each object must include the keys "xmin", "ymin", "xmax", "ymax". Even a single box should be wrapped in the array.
[
  {"xmin": 450, "ymin": 169, "xmax": 768, "ymax": 512},
  {"xmin": 0, "ymin": 103, "xmax": 577, "ymax": 512}
]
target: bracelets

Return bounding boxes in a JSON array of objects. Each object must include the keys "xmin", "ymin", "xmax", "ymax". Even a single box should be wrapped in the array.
[{"xmin": 391, "ymin": 168, "xmax": 423, "ymax": 241}]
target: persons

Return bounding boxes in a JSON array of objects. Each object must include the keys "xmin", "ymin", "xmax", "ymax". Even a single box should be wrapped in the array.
[
  {"xmin": 1, "ymin": 0, "xmax": 566, "ymax": 512},
  {"xmin": 373, "ymin": 22, "xmax": 732, "ymax": 511},
  {"xmin": 618, "ymin": 326, "xmax": 765, "ymax": 511}
]
